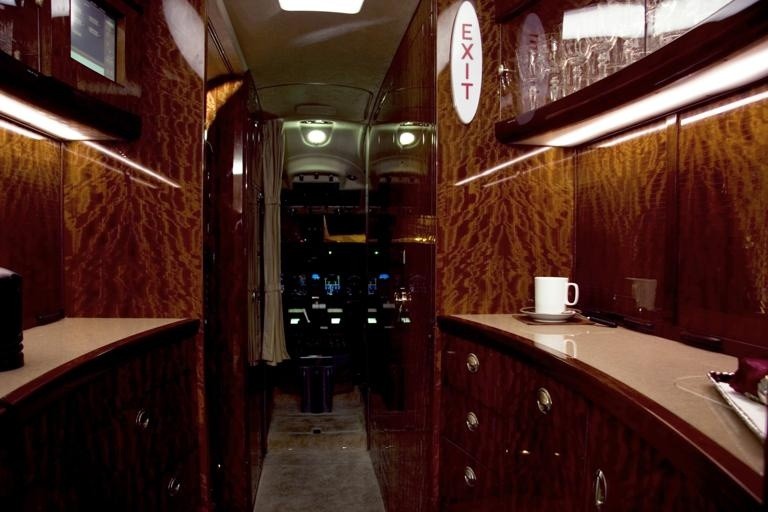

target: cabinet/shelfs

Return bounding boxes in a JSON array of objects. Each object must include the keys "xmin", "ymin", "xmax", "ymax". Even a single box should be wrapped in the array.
[
  {"xmin": 1, "ymin": 334, "xmax": 199, "ymax": 511},
  {"xmin": 437, "ymin": 331, "xmax": 768, "ymax": 510}
]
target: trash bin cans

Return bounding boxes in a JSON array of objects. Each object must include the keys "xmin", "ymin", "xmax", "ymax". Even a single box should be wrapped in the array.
[{"xmin": 299, "ymin": 356, "xmax": 334, "ymax": 413}]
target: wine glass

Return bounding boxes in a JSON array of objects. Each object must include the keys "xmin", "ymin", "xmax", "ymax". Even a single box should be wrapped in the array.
[{"xmin": 514, "ymin": 0, "xmax": 680, "ymax": 111}]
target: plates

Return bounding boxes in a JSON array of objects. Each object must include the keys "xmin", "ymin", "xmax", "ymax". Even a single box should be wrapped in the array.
[{"xmin": 517, "ymin": 306, "xmax": 581, "ymax": 325}]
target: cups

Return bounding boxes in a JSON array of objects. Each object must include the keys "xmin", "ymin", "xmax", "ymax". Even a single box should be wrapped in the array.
[{"xmin": 534, "ymin": 275, "xmax": 578, "ymax": 314}]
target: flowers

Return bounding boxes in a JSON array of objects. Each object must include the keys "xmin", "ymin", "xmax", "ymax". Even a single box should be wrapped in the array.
[{"xmin": 731, "ymin": 352, "xmax": 768, "ymax": 407}]
